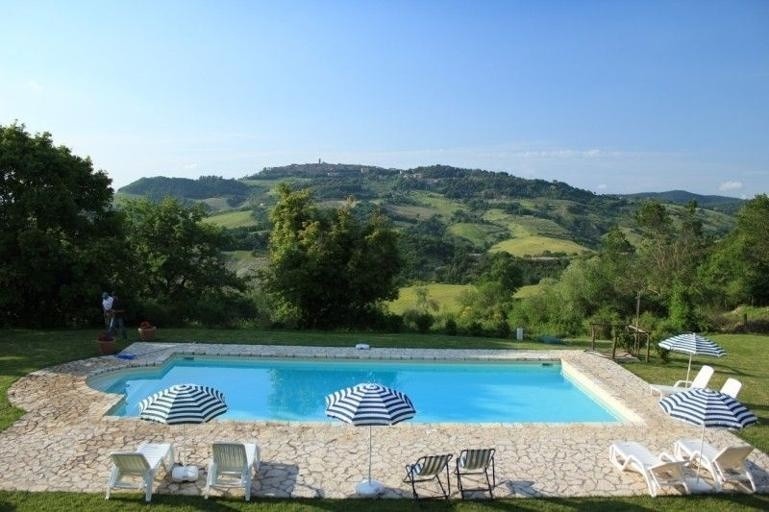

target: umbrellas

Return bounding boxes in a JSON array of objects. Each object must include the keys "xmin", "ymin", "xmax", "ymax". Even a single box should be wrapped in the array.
[
  {"xmin": 324, "ymin": 383, "xmax": 416, "ymax": 485},
  {"xmin": 658, "ymin": 387, "xmax": 760, "ymax": 484},
  {"xmin": 657, "ymin": 332, "xmax": 728, "ymax": 387},
  {"xmin": 137, "ymin": 383, "xmax": 228, "ymax": 466}
]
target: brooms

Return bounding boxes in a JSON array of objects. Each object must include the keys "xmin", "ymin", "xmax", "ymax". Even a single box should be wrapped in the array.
[{"xmin": 116, "ymin": 345, "xmax": 175, "ymax": 359}]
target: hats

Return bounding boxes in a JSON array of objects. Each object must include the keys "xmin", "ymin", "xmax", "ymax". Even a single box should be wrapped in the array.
[
  {"xmin": 102, "ymin": 293, "xmax": 108, "ymax": 298},
  {"xmin": 112, "ymin": 291, "xmax": 118, "ymax": 296}
]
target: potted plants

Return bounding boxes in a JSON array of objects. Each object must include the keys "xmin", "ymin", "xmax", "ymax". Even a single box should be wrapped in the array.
[
  {"xmin": 96, "ymin": 329, "xmax": 117, "ymax": 354},
  {"xmin": 137, "ymin": 321, "xmax": 157, "ymax": 340}
]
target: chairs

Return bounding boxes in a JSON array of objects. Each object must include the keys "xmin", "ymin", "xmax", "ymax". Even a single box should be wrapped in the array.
[
  {"xmin": 105, "ymin": 440, "xmax": 260, "ymax": 503},
  {"xmin": 403, "ymin": 447, "xmax": 499, "ymax": 502},
  {"xmin": 608, "ymin": 440, "xmax": 757, "ymax": 497},
  {"xmin": 650, "ymin": 364, "xmax": 743, "ymax": 400}
]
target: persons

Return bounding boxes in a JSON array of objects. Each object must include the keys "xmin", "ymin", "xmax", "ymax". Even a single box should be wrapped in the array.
[{"xmin": 102, "ymin": 292, "xmax": 114, "ymax": 328}]
